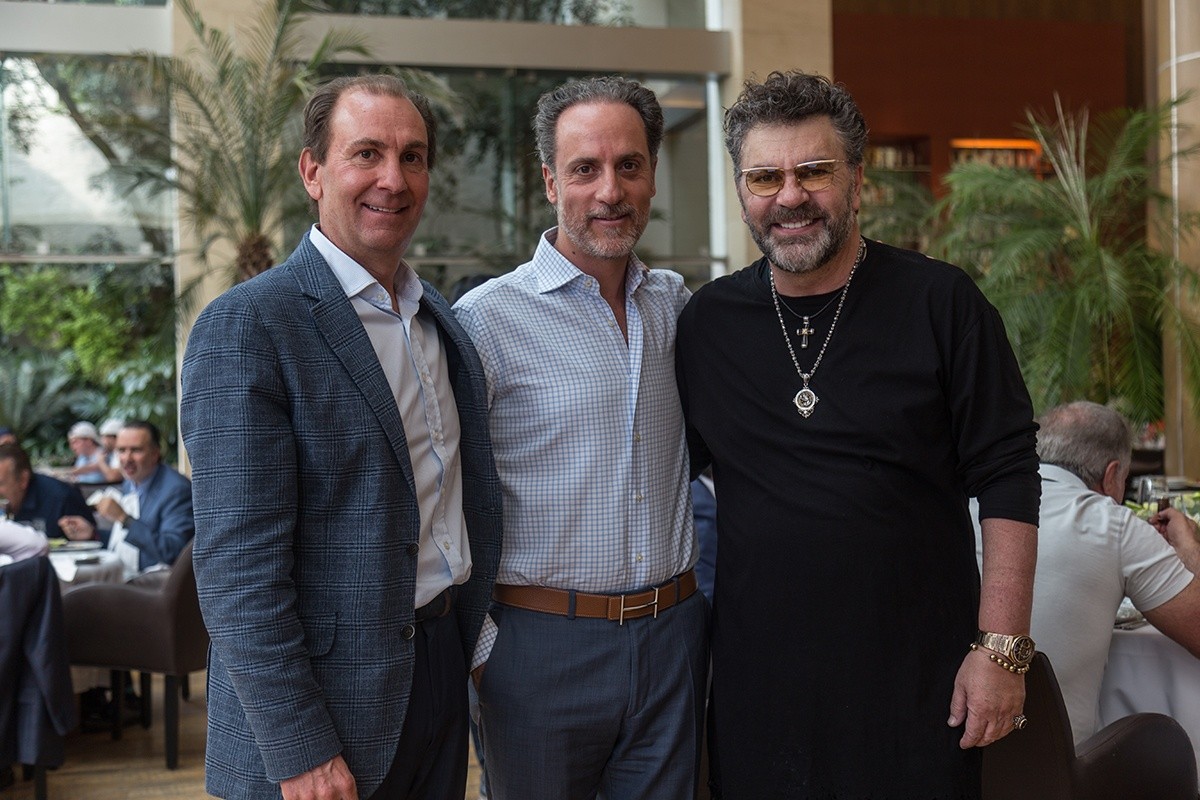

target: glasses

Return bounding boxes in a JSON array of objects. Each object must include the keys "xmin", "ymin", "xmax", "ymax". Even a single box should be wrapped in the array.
[{"xmin": 738, "ymin": 159, "xmax": 851, "ymax": 196}]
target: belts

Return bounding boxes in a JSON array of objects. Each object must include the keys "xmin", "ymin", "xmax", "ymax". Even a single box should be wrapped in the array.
[
  {"xmin": 491, "ymin": 566, "xmax": 699, "ymax": 626},
  {"xmin": 416, "ymin": 585, "xmax": 456, "ymax": 624}
]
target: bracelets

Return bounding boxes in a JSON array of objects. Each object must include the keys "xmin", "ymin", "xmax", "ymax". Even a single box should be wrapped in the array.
[{"xmin": 970, "ymin": 642, "xmax": 1030, "ymax": 674}]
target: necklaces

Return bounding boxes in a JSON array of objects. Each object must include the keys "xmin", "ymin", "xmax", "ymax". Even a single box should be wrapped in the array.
[{"xmin": 769, "ymin": 237, "xmax": 864, "ymax": 418}]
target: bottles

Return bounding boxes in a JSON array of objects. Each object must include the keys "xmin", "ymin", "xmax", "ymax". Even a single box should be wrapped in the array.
[
  {"xmin": 1156, "ymin": 495, "xmax": 1170, "ymax": 513},
  {"xmin": 0, "ymin": 499, "xmax": 16, "ymax": 521}
]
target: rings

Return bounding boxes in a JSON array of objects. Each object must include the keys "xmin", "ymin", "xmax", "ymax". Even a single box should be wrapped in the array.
[{"xmin": 1012, "ymin": 714, "xmax": 1028, "ymax": 729}]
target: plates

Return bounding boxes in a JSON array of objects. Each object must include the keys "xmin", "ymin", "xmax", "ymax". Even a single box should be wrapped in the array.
[
  {"xmin": 48, "ymin": 540, "xmax": 103, "ymax": 552},
  {"xmin": 1131, "ymin": 478, "xmax": 1200, "ymax": 490}
]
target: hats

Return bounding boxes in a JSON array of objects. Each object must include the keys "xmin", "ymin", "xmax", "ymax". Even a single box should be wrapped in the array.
[
  {"xmin": 67, "ymin": 421, "xmax": 101, "ymax": 445},
  {"xmin": 99, "ymin": 418, "xmax": 125, "ymax": 436}
]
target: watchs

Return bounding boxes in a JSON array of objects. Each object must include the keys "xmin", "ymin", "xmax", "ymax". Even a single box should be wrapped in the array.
[{"xmin": 975, "ymin": 631, "xmax": 1037, "ymax": 668}]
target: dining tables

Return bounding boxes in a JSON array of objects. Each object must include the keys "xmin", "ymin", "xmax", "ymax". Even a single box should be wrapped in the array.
[
  {"xmin": 0, "ymin": 543, "xmax": 119, "ymax": 733},
  {"xmin": 1092, "ymin": 624, "xmax": 1200, "ymax": 790}
]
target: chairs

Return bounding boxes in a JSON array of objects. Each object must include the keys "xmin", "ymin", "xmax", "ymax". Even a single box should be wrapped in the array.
[
  {"xmin": 0, "ymin": 555, "xmax": 72, "ymax": 800},
  {"xmin": 59, "ymin": 534, "xmax": 211, "ymax": 769},
  {"xmin": 979, "ymin": 650, "xmax": 1200, "ymax": 800}
]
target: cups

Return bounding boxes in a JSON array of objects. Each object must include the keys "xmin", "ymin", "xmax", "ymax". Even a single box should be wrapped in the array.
[
  {"xmin": 1138, "ymin": 476, "xmax": 1169, "ymax": 515},
  {"xmin": 1148, "ymin": 491, "xmax": 1186, "ymax": 517}
]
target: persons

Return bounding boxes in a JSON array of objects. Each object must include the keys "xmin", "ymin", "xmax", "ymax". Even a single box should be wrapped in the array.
[
  {"xmin": 0, "ymin": 416, "xmax": 196, "ymax": 734},
  {"xmin": 969, "ymin": 400, "xmax": 1200, "ymax": 750},
  {"xmin": 672, "ymin": 71, "xmax": 1042, "ymax": 800},
  {"xmin": 685, "ymin": 466, "xmax": 722, "ymax": 602},
  {"xmin": 181, "ymin": 75, "xmax": 503, "ymax": 800},
  {"xmin": 450, "ymin": 77, "xmax": 711, "ymax": 800}
]
